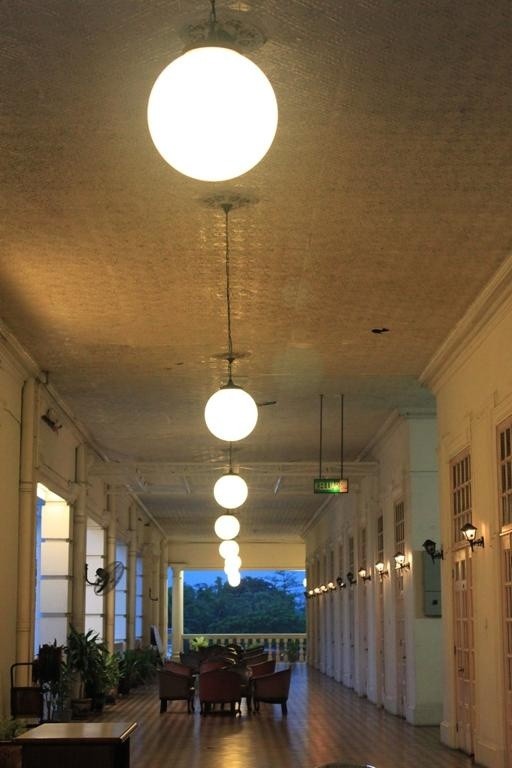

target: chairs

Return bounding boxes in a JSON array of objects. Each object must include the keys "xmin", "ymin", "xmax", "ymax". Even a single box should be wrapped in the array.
[
  {"xmin": 9, "ymin": 662, "xmax": 44, "ymax": 728},
  {"xmin": 157, "ymin": 637, "xmax": 293, "ymax": 722}
]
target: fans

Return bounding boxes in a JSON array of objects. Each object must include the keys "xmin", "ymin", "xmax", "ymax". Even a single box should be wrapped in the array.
[{"xmin": 85, "ymin": 559, "xmax": 129, "ymax": 601}]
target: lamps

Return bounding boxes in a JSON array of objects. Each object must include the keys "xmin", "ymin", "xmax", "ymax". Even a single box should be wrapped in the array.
[
  {"xmin": 460, "ymin": 522, "xmax": 485, "ymax": 552},
  {"xmin": 422, "ymin": 537, "xmax": 444, "ymax": 564},
  {"xmin": 212, "ymin": 441, "xmax": 250, "ymax": 509},
  {"xmin": 303, "ymin": 552, "xmax": 411, "ymax": 601},
  {"xmin": 204, "ymin": 195, "xmax": 259, "ymax": 441},
  {"xmin": 211, "ymin": 510, "xmax": 249, "ymax": 587},
  {"xmin": 146, "ymin": 0, "xmax": 279, "ymax": 184}
]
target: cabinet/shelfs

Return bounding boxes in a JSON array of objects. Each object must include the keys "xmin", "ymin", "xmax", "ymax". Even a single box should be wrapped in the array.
[{"xmin": 12, "ymin": 718, "xmax": 139, "ymax": 766}]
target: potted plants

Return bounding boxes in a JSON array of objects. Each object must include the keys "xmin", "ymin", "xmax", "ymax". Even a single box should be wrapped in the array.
[
  {"xmin": 37, "ymin": 630, "xmax": 156, "ymax": 720},
  {"xmin": 0, "ymin": 716, "xmax": 41, "ymax": 767}
]
